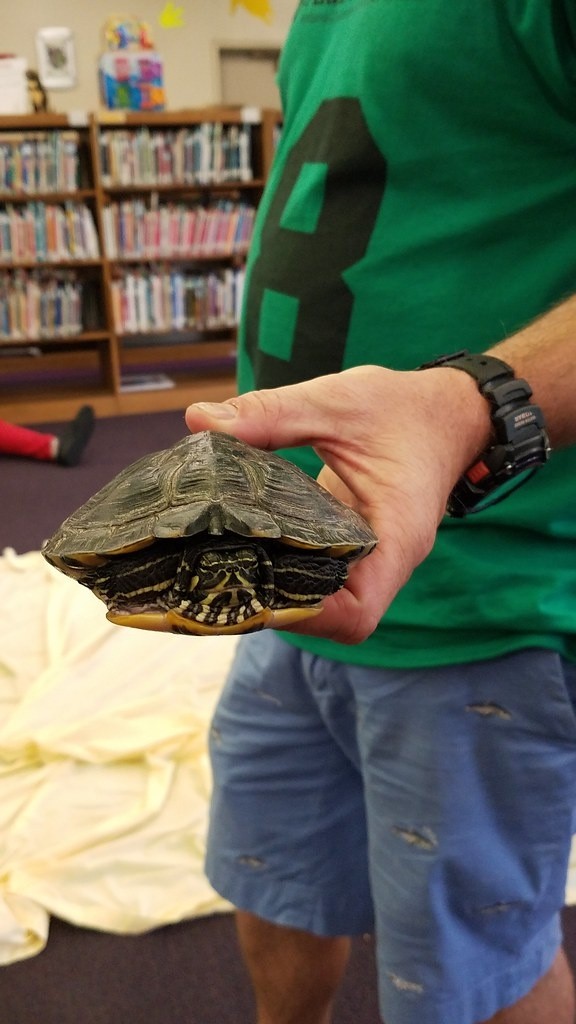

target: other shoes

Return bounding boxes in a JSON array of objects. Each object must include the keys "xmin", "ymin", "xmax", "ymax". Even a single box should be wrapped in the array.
[{"xmin": 55, "ymin": 405, "xmax": 95, "ymax": 467}]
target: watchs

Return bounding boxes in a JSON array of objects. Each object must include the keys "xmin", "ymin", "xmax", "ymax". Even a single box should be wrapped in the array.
[{"xmin": 415, "ymin": 352, "xmax": 551, "ymax": 520}]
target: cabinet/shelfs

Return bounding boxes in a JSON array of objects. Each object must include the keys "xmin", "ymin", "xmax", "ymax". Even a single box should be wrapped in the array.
[{"xmin": 1, "ymin": 107, "xmax": 284, "ymax": 425}]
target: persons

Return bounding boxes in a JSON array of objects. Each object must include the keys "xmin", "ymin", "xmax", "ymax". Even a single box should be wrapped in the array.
[
  {"xmin": 184, "ymin": 0, "xmax": 576, "ymax": 1024},
  {"xmin": 0, "ymin": 406, "xmax": 95, "ymax": 465}
]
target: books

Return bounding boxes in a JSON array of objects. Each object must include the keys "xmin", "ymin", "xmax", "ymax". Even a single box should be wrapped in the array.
[{"xmin": 0, "ymin": 122, "xmax": 263, "ymax": 345}]
